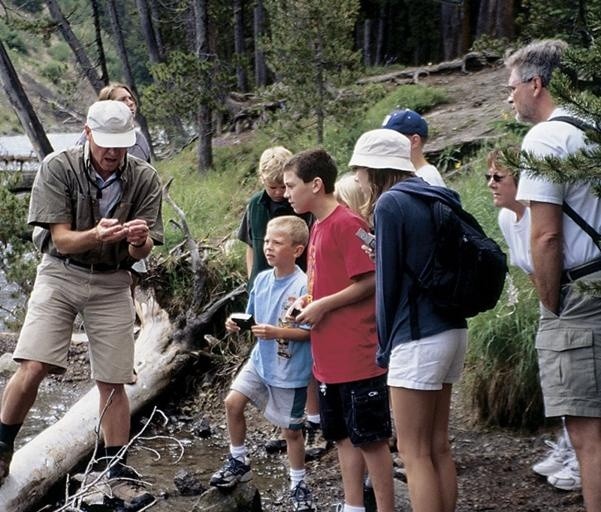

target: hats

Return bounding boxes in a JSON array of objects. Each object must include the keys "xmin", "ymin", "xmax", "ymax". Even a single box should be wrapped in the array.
[
  {"xmin": 349, "ymin": 127, "xmax": 416, "ymax": 174},
  {"xmin": 382, "ymin": 110, "xmax": 429, "ymax": 140},
  {"xmin": 85, "ymin": 100, "xmax": 140, "ymax": 151}
]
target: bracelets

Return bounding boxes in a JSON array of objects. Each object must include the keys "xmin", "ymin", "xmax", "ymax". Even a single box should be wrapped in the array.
[{"xmin": 129, "ymin": 240, "xmax": 147, "ymax": 248}]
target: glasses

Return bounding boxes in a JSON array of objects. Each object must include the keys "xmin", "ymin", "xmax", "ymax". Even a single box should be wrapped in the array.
[{"xmin": 483, "ymin": 172, "xmax": 510, "ymax": 186}]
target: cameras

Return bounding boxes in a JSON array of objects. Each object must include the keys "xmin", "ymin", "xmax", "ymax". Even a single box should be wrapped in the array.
[{"xmin": 231, "ymin": 313, "xmax": 257, "ymax": 330}]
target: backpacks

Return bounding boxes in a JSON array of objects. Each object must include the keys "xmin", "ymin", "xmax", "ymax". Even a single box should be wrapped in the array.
[{"xmin": 391, "ymin": 180, "xmax": 505, "ymax": 322}]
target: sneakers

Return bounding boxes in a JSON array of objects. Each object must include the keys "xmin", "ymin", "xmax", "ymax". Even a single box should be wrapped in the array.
[
  {"xmin": 210, "ymin": 454, "xmax": 255, "ymax": 488},
  {"xmin": 547, "ymin": 449, "xmax": 586, "ymax": 491},
  {"xmin": 532, "ymin": 437, "xmax": 572, "ymax": 476},
  {"xmin": 101, "ymin": 470, "xmax": 155, "ymax": 509},
  {"xmin": 302, "ymin": 417, "xmax": 331, "ymax": 459},
  {"xmin": 0, "ymin": 440, "xmax": 16, "ymax": 487},
  {"xmin": 287, "ymin": 481, "xmax": 316, "ymax": 508},
  {"xmin": 266, "ymin": 425, "xmax": 288, "ymax": 453}
]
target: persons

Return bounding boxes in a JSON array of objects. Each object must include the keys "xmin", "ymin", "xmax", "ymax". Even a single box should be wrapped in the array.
[
  {"xmin": 279, "ymin": 147, "xmax": 397, "ymax": 511},
  {"xmin": 487, "ymin": 146, "xmax": 535, "ymax": 288},
  {"xmin": 334, "ymin": 174, "xmax": 375, "ymax": 228},
  {"xmin": 502, "ymin": 38, "xmax": 601, "ymax": 511},
  {"xmin": 10, "ymin": 99, "xmax": 165, "ymax": 506},
  {"xmin": 207, "ymin": 215, "xmax": 315, "ymax": 511},
  {"xmin": 236, "ymin": 146, "xmax": 313, "ymax": 301},
  {"xmin": 382, "ymin": 108, "xmax": 445, "ymax": 188},
  {"xmin": 349, "ymin": 129, "xmax": 469, "ymax": 512},
  {"xmin": 75, "ymin": 82, "xmax": 151, "ymax": 328}
]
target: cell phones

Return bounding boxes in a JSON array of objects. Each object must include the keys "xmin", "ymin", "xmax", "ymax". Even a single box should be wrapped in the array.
[
  {"xmin": 355, "ymin": 228, "xmax": 376, "ymax": 250},
  {"xmin": 291, "ymin": 308, "xmax": 302, "ymax": 318}
]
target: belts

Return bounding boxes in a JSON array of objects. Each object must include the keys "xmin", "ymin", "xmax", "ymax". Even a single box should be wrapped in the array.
[
  {"xmin": 45, "ymin": 247, "xmax": 133, "ymax": 276},
  {"xmin": 556, "ymin": 260, "xmax": 600, "ymax": 284}
]
